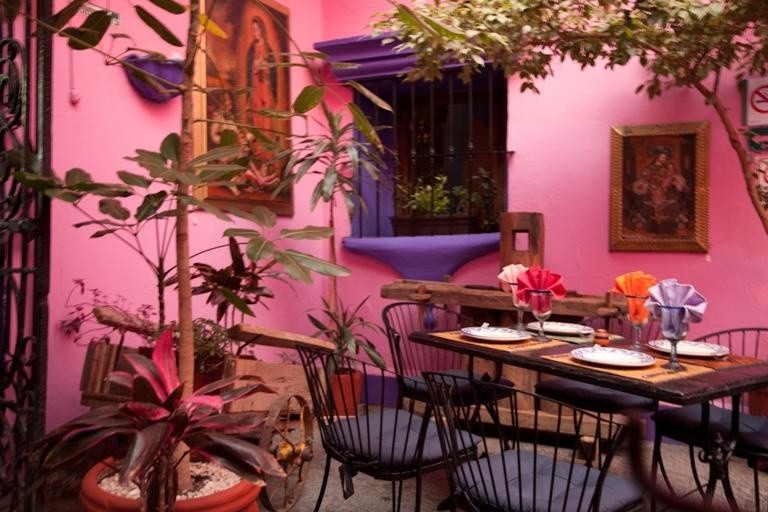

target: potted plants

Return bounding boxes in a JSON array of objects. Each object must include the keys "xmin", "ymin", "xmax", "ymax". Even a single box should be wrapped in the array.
[
  {"xmin": 389, "ymin": 167, "xmax": 496, "ymax": 236},
  {"xmin": 34, "ymin": 324, "xmax": 286, "ymax": 511},
  {"xmin": 308, "ymin": 293, "xmax": 384, "ymax": 416}
]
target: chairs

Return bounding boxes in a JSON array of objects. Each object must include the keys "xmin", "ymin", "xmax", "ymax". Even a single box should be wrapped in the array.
[
  {"xmin": 294, "ymin": 343, "xmax": 481, "ymax": 510},
  {"xmin": 381, "ymin": 302, "xmax": 518, "ymax": 461},
  {"xmin": 654, "ymin": 324, "xmax": 767, "ymax": 510},
  {"xmin": 420, "ymin": 368, "xmax": 646, "ymax": 511},
  {"xmin": 525, "ymin": 282, "xmax": 678, "ymax": 502}
]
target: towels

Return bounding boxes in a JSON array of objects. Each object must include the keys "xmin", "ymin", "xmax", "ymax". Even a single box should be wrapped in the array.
[
  {"xmin": 496, "ymin": 264, "xmax": 531, "ymax": 306},
  {"xmin": 644, "ymin": 277, "xmax": 706, "ymax": 335},
  {"xmin": 513, "ymin": 263, "xmax": 567, "ymax": 315},
  {"xmin": 616, "ymin": 271, "xmax": 666, "ymax": 324}
]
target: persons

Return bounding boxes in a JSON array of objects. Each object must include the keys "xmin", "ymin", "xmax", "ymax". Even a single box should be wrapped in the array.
[
  {"xmin": 246, "ymin": 16, "xmax": 276, "ymax": 159},
  {"xmin": 644, "ymin": 150, "xmax": 666, "ymax": 177},
  {"xmin": 660, "ymin": 162, "xmax": 688, "ymax": 199}
]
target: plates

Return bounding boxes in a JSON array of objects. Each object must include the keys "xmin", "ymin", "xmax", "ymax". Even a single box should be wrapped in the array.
[
  {"xmin": 459, "ymin": 324, "xmax": 532, "ymax": 342},
  {"xmin": 570, "ymin": 347, "xmax": 657, "ymax": 367},
  {"xmin": 526, "ymin": 320, "xmax": 595, "ymax": 336},
  {"xmin": 646, "ymin": 338, "xmax": 733, "ymax": 357}
]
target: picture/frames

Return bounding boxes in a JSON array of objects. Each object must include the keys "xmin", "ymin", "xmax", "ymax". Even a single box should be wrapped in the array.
[
  {"xmin": 607, "ymin": 122, "xmax": 713, "ymax": 254},
  {"xmin": 190, "ymin": 0, "xmax": 296, "ymax": 218}
]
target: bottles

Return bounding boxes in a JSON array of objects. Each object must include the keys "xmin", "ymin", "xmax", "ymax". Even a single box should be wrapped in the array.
[{"xmin": 592, "ymin": 327, "xmax": 608, "ymax": 347}]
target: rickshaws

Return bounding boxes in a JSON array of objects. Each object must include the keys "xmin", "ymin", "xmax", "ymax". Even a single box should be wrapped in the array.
[{"xmin": 79, "ymin": 303, "xmax": 337, "ymax": 511}]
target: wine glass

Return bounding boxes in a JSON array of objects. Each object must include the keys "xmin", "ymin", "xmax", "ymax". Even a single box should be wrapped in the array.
[
  {"xmin": 511, "ymin": 282, "xmax": 530, "ymax": 330},
  {"xmin": 656, "ymin": 304, "xmax": 689, "ymax": 373},
  {"xmin": 624, "ymin": 295, "xmax": 652, "ymax": 349},
  {"xmin": 526, "ymin": 289, "xmax": 552, "ymax": 343}
]
